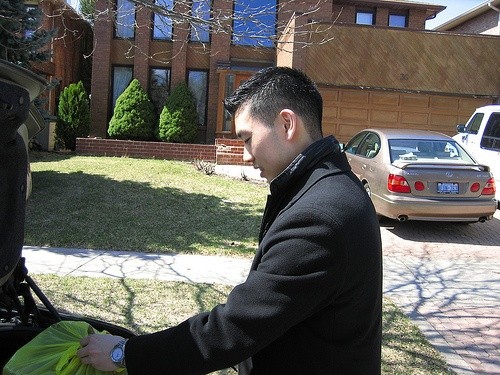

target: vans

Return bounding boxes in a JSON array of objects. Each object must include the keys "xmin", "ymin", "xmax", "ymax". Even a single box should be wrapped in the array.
[{"xmin": 450, "ymin": 105, "xmax": 500, "ymax": 203}]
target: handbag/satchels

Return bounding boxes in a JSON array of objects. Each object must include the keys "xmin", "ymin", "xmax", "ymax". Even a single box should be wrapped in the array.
[{"xmin": 2, "ymin": 321, "xmax": 128, "ymax": 375}]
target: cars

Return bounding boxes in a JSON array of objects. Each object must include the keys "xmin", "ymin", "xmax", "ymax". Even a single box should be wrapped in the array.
[
  {"xmin": 0, "ymin": 60, "xmax": 143, "ymax": 374},
  {"xmin": 338, "ymin": 128, "xmax": 497, "ymax": 223}
]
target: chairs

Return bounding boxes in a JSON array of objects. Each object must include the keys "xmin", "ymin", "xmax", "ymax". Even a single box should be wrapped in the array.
[
  {"xmin": 365, "ymin": 142, "xmax": 380, "ymax": 158},
  {"xmin": 433, "ymin": 142, "xmax": 450, "ymax": 157},
  {"xmin": 412, "ymin": 142, "xmax": 436, "ymax": 158}
]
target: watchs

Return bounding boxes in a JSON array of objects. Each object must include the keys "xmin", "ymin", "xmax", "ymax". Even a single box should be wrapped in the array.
[{"xmin": 110, "ymin": 339, "xmax": 127, "ymax": 368}]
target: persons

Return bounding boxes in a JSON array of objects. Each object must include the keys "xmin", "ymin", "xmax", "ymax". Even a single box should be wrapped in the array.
[{"xmin": 77, "ymin": 68, "xmax": 383, "ymax": 375}]
